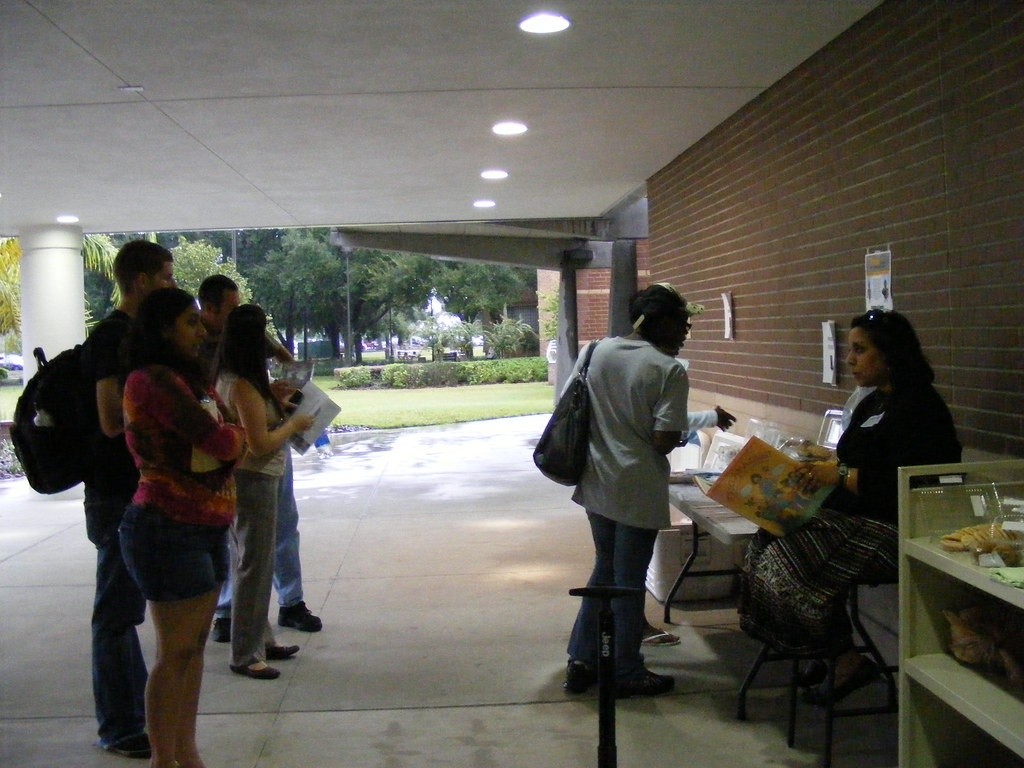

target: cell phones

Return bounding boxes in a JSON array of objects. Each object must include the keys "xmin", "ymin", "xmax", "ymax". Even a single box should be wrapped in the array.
[{"xmin": 285, "ymin": 389, "xmax": 304, "ymax": 414}]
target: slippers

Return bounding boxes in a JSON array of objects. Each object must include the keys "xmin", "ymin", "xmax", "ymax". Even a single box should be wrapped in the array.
[{"xmin": 641, "ymin": 630, "xmax": 682, "ymax": 647}]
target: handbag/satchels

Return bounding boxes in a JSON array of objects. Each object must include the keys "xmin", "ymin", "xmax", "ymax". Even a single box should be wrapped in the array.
[{"xmin": 532, "ymin": 336, "xmax": 596, "ymax": 485}]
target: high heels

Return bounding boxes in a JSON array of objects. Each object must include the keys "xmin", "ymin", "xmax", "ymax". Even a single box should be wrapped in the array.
[
  {"xmin": 614, "ymin": 662, "xmax": 673, "ymax": 699},
  {"xmin": 564, "ymin": 658, "xmax": 602, "ymax": 692}
]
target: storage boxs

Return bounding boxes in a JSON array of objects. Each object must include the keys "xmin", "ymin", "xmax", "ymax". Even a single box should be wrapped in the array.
[{"xmin": 645, "ymin": 531, "xmax": 734, "ymax": 601}]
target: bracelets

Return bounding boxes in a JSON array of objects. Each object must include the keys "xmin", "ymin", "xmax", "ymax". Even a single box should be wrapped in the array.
[{"xmin": 844, "ymin": 468, "xmax": 851, "ymax": 491}]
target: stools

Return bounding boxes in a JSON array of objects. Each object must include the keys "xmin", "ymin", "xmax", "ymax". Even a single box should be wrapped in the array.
[{"xmin": 788, "ymin": 582, "xmax": 898, "ymax": 768}]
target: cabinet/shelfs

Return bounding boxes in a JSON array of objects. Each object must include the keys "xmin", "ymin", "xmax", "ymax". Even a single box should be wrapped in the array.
[{"xmin": 896, "ymin": 460, "xmax": 1024, "ymax": 768}]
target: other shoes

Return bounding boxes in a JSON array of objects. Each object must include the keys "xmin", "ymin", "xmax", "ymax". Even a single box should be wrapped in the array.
[
  {"xmin": 802, "ymin": 656, "xmax": 879, "ymax": 707},
  {"xmin": 265, "ymin": 644, "xmax": 299, "ymax": 658},
  {"xmin": 230, "ymin": 659, "xmax": 280, "ymax": 679},
  {"xmin": 108, "ymin": 734, "xmax": 152, "ymax": 760},
  {"xmin": 793, "ymin": 664, "xmax": 827, "ymax": 688}
]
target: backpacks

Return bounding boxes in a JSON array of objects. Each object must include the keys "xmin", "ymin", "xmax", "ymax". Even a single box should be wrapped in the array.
[{"xmin": 9, "ymin": 313, "xmax": 133, "ymax": 494}]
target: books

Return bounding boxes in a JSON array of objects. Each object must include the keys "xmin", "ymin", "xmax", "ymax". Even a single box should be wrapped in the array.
[{"xmin": 694, "ymin": 436, "xmax": 836, "ymax": 537}]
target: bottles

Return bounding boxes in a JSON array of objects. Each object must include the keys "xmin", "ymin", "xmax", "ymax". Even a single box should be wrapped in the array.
[
  {"xmin": 33, "ymin": 347, "xmax": 47, "ymax": 370},
  {"xmin": 314, "ymin": 431, "xmax": 334, "ymax": 459},
  {"xmin": 32, "ymin": 401, "xmax": 54, "ymax": 427}
]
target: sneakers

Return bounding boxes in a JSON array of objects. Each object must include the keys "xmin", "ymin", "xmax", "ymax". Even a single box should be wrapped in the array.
[
  {"xmin": 279, "ymin": 601, "xmax": 322, "ymax": 632},
  {"xmin": 212, "ymin": 617, "xmax": 232, "ymax": 643}
]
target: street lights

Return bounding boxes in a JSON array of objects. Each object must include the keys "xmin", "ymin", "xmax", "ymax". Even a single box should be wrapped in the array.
[{"xmin": 340, "ymin": 246, "xmax": 354, "ymax": 366}]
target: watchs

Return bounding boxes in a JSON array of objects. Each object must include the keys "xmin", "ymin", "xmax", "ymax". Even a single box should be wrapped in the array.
[{"xmin": 838, "ymin": 463, "xmax": 847, "ymax": 490}]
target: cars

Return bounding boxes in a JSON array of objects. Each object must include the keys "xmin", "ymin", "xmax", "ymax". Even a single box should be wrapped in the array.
[{"xmin": 0, "ymin": 355, "xmax": 23, "ymax": 370}]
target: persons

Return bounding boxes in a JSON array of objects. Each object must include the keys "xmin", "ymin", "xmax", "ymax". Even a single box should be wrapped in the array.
[
  {"xmin": 737, "ymin": 309, "xmax": 960, "ymax": 707},
  {"xmin": 119, "ymin": 287, "xmax": 245, "ymax": 768},
  {"xmin": 561, "ymin": 283, "xmax": 693, "ymax": 698},
  {"xmin": 197, "ymin": 274, "xmax": 323, "ymax": 679},
  {"xmin": 84, "ymin": 239, "xmax": 179, "ymax": 758},
  {"xmin": 641, "ymin": 344, "xmax": 738, "ymax": 647}
]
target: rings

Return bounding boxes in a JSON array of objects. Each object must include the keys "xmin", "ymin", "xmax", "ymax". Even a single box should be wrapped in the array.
[{"xmin": 809, "ymin": 473, "xmax": 812, "ymax": 479}]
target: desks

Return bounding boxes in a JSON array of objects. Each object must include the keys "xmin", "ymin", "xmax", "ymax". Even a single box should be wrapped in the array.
[{"xmin": 665, "ymin": 480, "xmax": 762, "ymax": 720}]
